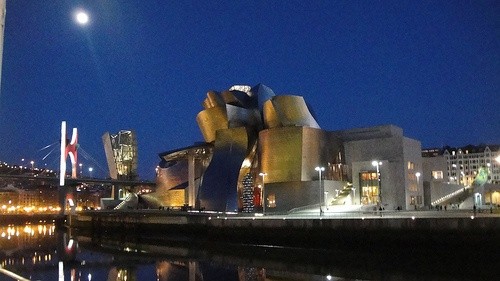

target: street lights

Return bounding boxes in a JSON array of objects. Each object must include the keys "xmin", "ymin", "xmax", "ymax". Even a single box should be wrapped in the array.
[
  {"xmin": 415, "ymin": 171, "xmax": 422, "ymax": 212},
  {"xmin": 259, "ymin": 172, "xmax": 268, "ymax": 213},
  {"xmin": 315, "ymin": 166, "xmax": 325, "ymax": 216},
  {"xmin": 371, "ymin": 160, "xmax": 381, "ymax": 215}
]
